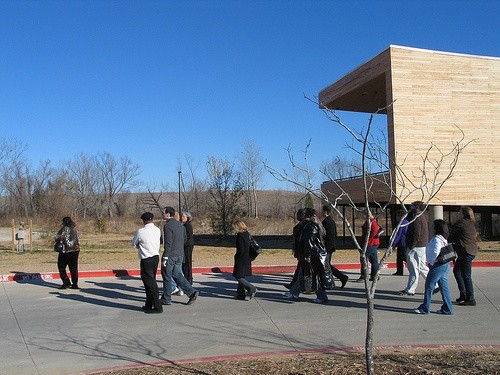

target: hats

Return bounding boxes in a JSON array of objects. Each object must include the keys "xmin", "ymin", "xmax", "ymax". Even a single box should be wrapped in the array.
[{"xmin": 140, "ymin": 212, "xmax": 154, "ymax": 220}]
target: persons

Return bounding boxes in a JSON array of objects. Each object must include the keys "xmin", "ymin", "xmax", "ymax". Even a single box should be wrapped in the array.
[
  {"xmin": 132, "ymin": 207, "xmax": 199, "ymax": 311},
  {"xmin": 283, "ymin": 208, "xmax": 328, "ymax": 303},
  {"xmin": 413, "ymin": 220, "xmax": 453, "ymax": 315},
  {"xmin": 54, "ymin": 217, "xmax": 80, "ymax": 288},
  {"xmin": 231, "ymin": 219, "xmax": 257, "ymax": 300},
  {"xmin": 448, "ymin": 206, "xmax": 478, "ymax": 306},
  {"xmin": 356, "ymin": 209, "xmax": 380, "ymax": 282},
  {"xmin": 392, "ymin": 210, "xmax": 408, "ymax": 276},
  {"xmin": 320, "ymin": 205, "xmax": 349, "ymax": 288},
  {"xmin": 399, "ymin": 201, "xmax": 440, "ymax": 296},
  {"xmin": 18, "ymin": 222, "xmax": 25, "ymax": 252}
]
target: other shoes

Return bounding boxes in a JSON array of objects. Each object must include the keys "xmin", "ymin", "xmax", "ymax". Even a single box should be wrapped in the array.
[
  {"xmin": 398, "ymin": 290, "xmax": 415, "ymax": 297},
  {"xmin": 59, "ymin": 283, "xmax": 79, "ymax": 289},
  {"xmin": 340, "ymin": 274, "xmax": 349, "ymax": 287},
  {"xmin": 413, "ymin": 307, "xmax": 425, "ymax": 313},
  {"xmin": 250, "ymin": 288, "xmax": 257, "ymax": 300},
  {"xmin": 283, "ymin": 283, "xmax": 292, "ymax": 290},
  {"xmin": 437, "ymin": 309, "xmax": 443, "ymax": 314},
  {"xmin": 456, "ymin": 297, "xmax": 476, "ymax": 306},
  {"xmin": 231, "ymin": 296, "xmax": 244, "ymax": 300},
  {"xmin": 312, "ymin": 299, "xmax": 326, "ymax": 303},
  {"xmin": 280, "ymin": 293, "xmax": 299, "ymax": 301},
  {"xmin": 139, "ymin": 306, "xmax": 162, "ymax": 313},
  {"xmin": 171, "ymin": 287, "xmax": 184, "ymax": 296},
  {"xmin": 186, "ymin": 291, "xmax": 199, "ymax": 305},
  {"xmin": 432, "ymin": 287, "xmax": 441, "ymax": 294}
]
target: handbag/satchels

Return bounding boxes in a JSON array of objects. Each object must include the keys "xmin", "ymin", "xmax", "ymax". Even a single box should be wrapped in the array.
[
  {"xmin": 248, "ymin": 232, "xmax": 262, "ymax": 261},
  {"xmin": 435, "ymin": 236, "xmax": 456, "ymax": 266},
  {"xmin": 371, "ymin": 220, "xmax": 386, "ymax": 239},
  {"xmin": 304, "ymin": 262, "xmax": 324, "ymax": 293},
  {"xmin": 54, "ymin": 239, "xmax": 64, "ymax": 252}
]
